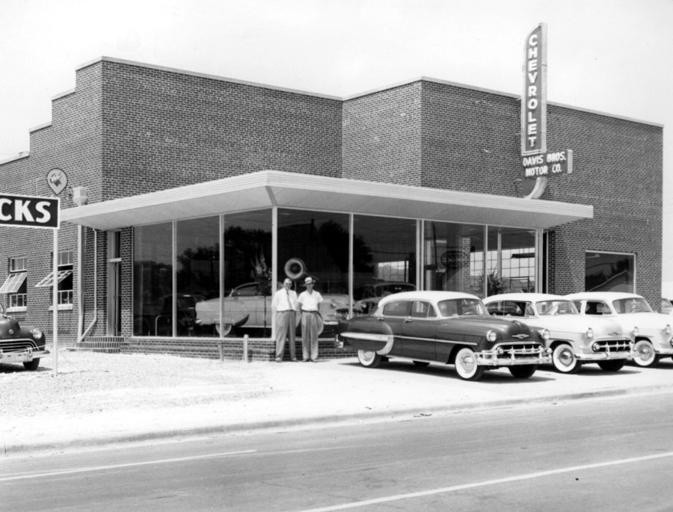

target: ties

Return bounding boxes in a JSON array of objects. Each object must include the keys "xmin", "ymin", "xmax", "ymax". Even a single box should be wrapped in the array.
[{"xmin": 286, "ymin": 290, "xmax": 293, "ymax": 310}]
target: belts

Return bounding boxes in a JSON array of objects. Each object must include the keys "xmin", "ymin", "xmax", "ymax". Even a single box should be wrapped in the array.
[
  {"xmin": 301, "ymin": 310, "xmax": 317, "ymax": 314},
  {"xmin": 276, "ymin": 310, "xmax": 296, "ymax": 313}
]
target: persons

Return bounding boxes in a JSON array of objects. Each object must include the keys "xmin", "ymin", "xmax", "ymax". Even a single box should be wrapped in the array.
[
  {"xmin": 297, "ymin": 276, "xmax": 324, "ymax": 362},
  {"xmin": 270, "ymin": 278, "xmax": 300, "ymax": 361}
]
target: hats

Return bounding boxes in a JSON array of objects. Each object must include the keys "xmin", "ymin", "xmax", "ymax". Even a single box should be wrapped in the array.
[{"xmin": 302, "ymin": 276, "xmax": 316, "ymax": 286}]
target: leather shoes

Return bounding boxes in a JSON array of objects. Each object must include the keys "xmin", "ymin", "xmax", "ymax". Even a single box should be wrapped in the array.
[
  {"xmin": 291, "ymin": 358, "xmax": 298, "ymax": 361},
  {"xmin": 275, "ymin": 357, "xmax": 283, "ymax": 362},
  {"xmin": 302, "ymin": 358, "xmax": 318, "ymax": 362}
]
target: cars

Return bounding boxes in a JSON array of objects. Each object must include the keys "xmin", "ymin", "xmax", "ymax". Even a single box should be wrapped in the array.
[
  {"xmin": 0, "ymin": 302, "xmax": 50, "ymax": 370},
  {"xmin": 160, "ymin": 293, "xmax": 206, "ymax": 335}
]
